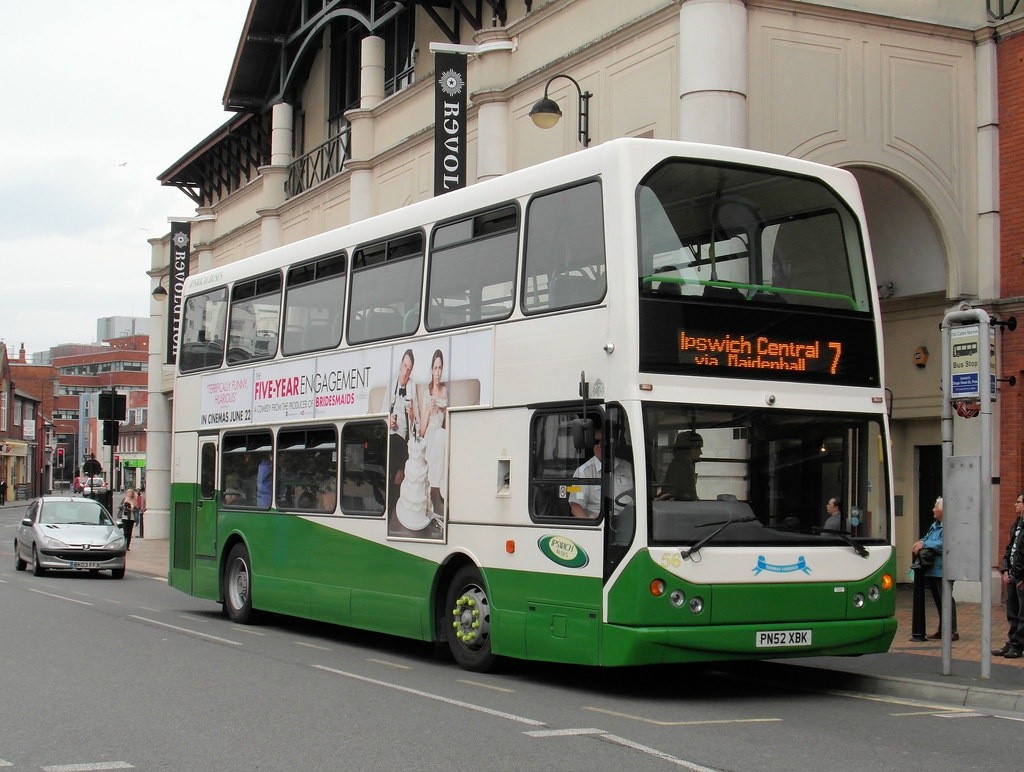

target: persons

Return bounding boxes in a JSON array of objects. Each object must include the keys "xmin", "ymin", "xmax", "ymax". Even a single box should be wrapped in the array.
[
  {"xmin": 421, "ymin": 347, "xmax": 448, "ymax": 496},
  {"xmin": 567, "ymin": 428, "xmax": 636, "ymax": 520},
  {"xmin": 136, "ymin": 487, "xmax": 147, "ymax": 539},
  {"xmin": 911, "ymin": 496, "xmax": 962, "ymax": 641},
  {"xmin": 990, "ymin": 493, "xmax": 1024, "ymax": 659},
  {"xmin": 118, "ymin": 488, "xmax": 139, "ymax": 552},
  {"xmin": 379, "ymin": 349, "xmax": 420, "ymax": 496},
  {"xmin": 0, "ymin": 478, "xmax": 7, "ymax": 505},
  {"xmin": 665, "ymin": 430, "xmax": 704, "ymax": 502},
  {"xmin": 819, "ymin": 496, "xmax": 842, "ymax": 538},
  {"xmin": 133, "ymin": 491, "xmax": 142, "ymax": 526},
  {"xmin": 203, "ymin": 444, "xmax": 368, "ymax": 511}
]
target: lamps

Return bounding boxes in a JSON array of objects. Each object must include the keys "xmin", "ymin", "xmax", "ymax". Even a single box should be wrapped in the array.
[{"xmin": 528, "ymin": 74, "xmax": 592, "ymax": 148}]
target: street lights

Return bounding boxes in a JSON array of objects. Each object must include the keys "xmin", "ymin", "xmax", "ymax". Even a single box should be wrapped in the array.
[
  {"xmin": 61, "ymin": 425, "xmax": 76, "ymax": 492},
  {"xmin": 114, "ymin": 455, "xmax": 119, "ymax": 471},
  {"xmin": 59, "ymin": 449, "xmax": 64, "ymax": 468}
]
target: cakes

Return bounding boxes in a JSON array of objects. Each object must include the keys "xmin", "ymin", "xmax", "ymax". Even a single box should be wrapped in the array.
[{"xmin": 396, "ymin": 435, "xmax": 431, "ymax": 527}]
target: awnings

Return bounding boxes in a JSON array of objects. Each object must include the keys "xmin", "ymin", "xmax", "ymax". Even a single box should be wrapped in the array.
[{"xmin": 123, "ymin": 459, "xmax": 147, "ymax": 488}]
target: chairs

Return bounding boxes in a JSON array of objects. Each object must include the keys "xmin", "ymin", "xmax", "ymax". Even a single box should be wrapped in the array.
[{"xmin": 180, "ymin": 266, "xmax": 790, "ymax": 372}]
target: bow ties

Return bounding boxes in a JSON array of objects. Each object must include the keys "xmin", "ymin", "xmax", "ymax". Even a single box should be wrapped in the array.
[{"xmin": 399, "ymin": 387, "xmax": 407, "ymax": 397}]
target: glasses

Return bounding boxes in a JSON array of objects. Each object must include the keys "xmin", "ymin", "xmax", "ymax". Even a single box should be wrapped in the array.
[{"xmin": 595, "ymin": 439, "xmax": 603, "ymax": 445}]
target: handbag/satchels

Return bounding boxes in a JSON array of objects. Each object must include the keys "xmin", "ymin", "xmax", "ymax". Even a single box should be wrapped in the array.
[
  {"xmin": 910, "ymin": 545, "xmax": 937, "ymax": 571},
  {"xmin": 117, "ymin": 508, "xmax": 122, "ymax": 518}
]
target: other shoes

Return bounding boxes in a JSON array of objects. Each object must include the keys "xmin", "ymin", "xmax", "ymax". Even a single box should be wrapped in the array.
[
  {"xmin": 927, "ymin": 632, "xmax": 942, "ymax": 639},
  {"xmin": 992, "ymin": 645, "xmax": 1010, "ymax": 656},
  {"xmin": 952, "ymin": 633, "xmax": 960, "ymax": 640},
  {"xmin": 1004, "ymin": 648, "xmax": 1022, "ymax": 657}
]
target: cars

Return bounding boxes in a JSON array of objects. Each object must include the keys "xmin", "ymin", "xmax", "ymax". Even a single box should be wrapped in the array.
[
  {"xmin": 73, "ymin": 476, "xmax": 88, "ymax": 494},
  {"xmin": 13, "ymin": 496, "xmax": 128, "ymax": 579},
  {"xmin": 79, "ymin": 478, "xmax": 108, "ymax": 498}
]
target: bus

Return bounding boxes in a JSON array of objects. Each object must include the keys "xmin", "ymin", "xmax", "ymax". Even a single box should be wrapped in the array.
[{"xmin": 165, "ymin": 136, "xmax": 899, "ymax": 674}]
